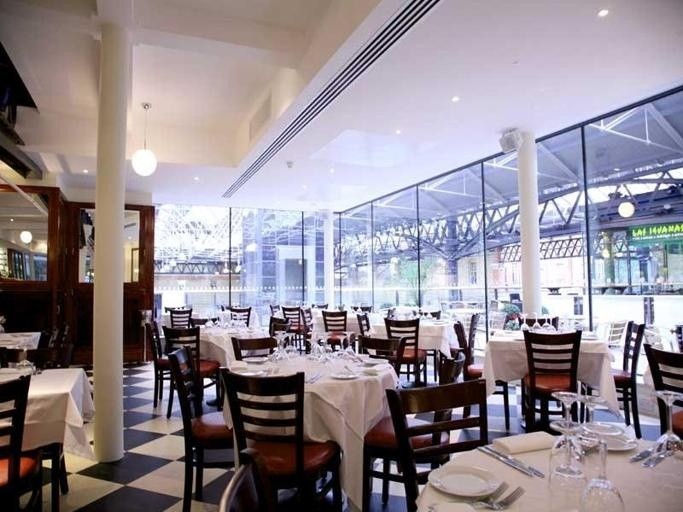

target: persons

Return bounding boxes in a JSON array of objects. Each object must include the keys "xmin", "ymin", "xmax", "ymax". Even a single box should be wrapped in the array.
[{"xmin": 501, "ymin": 304, "xmax": 524, "ymax": 330}]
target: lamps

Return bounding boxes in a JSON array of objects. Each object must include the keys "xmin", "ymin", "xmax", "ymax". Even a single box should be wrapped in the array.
[{"xmin": 129, "ymin": 101, "xmax": 157, "ymax": 178}]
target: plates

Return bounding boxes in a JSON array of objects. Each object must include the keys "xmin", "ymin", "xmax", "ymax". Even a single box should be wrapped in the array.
[{"xmin": 428, "ymin": 463, "xmax": 503, "ymax": 497}]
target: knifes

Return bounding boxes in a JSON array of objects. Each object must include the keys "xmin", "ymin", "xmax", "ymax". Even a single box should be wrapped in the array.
[{"xmin": 477, "ymin": 444, "xmax": 544, "ymax": 479}]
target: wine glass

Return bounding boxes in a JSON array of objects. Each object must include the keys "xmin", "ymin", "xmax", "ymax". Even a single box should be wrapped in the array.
[
  {"xmin": 496, "ymin": 311, "xmax": 602, "ymax": 341},
  {"xmin": 227, "ymin": 328, "xmax": 387, "ymax": 383},
  {"xmin": 550, "ymin": 389, "xmax": 682, "ymax": 512},
  {"xmin": 15, "ymin": 344, "xmax": 34, "ymax": 379}
]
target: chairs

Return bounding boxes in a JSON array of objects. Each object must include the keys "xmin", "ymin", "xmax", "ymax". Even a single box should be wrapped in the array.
[
  {"xmin": 0, "ymin": 319, "xmax": 73, "ymax": 368},
  {"xmin": 145, "ymin": 303, "xmax": 645, "ymax": 437},
  {"xmin": 644, "ymin": 344, "xmax": 682, "ymax": 437},
  {"xmin": 220, "ymin": 449, "xmax": 339, "ymax": 511},
  {"xmin": 385, "ymin": 378, "xmax": 488, "ymax": 511},
  {"xmin": 219, "ymin": 368, "xmax": 340, "ymax": 510},
  {"xmin": 360, "ymin": 348, "xmax": 466, "ymax": 509},
  {"xmin": 0, "ymin": 373, "xmax": 43, "ymax": 511},
  {"xmin": 167, "ymin": 345, "xmax": 234, "ymax": 511}
]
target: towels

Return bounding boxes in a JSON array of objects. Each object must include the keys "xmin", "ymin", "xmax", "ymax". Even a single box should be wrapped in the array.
[{"xmin": 496, "ymin": 430, "xmax": 556, "ymax": 453}]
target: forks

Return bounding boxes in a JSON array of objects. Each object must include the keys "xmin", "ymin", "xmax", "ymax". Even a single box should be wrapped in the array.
[{"xmin": 431, "ymin": 483, "xmax": 524, "ymax": 511}]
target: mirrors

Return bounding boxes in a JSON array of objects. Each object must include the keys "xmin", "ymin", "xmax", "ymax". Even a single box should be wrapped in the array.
[
  {"xmin": 79, "ymin": 208, "xmax": 140, "ymax": 282},
  {"xmin": 0, "ymin": 190, "xmax": 48, "ymax": 281}
]
